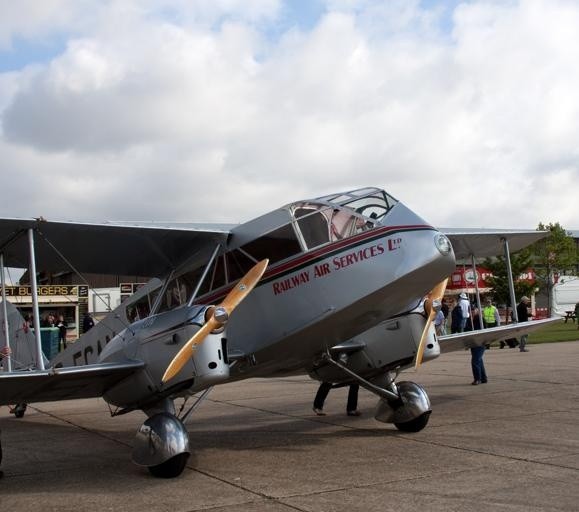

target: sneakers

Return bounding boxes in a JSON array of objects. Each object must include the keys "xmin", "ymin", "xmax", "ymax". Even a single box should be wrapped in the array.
[
  {"xmin": 500, "ymin": 343, "xmax": 505, "ymax": 349},
  {"xmin": 472, "ymin": 378, "xmax": 487, "ymax": 385},
  {"xmin": 520, "ymin": 348, "xmax": 529, "ymax": 352}
]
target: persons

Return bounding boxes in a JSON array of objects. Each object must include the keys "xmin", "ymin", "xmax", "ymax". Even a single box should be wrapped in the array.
[
  {"xmin": 512, "ymin": 294, "xmax": 534, "ymax": 353},
  {"xmin": 83, "ymin": 313, "xmax": 94, "ymax": 333},
  {"xmin": 463, "ymin": 302, "xmax": 488, "ymax": 385},
  {"xmin": 434, "ymin": 292, "xmax": 505, "ymax": 350},
  {"xmin": 311, "ymin": 381, "xmax": 364, "ymax": 418},
  {"xmin": 26, "ymin": 311, "xmax": 67, "ymax": 352}
]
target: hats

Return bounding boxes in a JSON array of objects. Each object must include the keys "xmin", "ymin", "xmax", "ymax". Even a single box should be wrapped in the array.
[
  {"xmin": 487, "ymin": 300, "xmax": 491, "ymax": 303},
  {"xmin": 459, "ymin": 293, "xmax": 468, "ymax": 299},
  {"xmin": 468, "ymin": 304, "xmax": 476, "ymax": 311},
  {"xmin": 521, "ymin": 296, "xmax": 528, "ymax": 302}
]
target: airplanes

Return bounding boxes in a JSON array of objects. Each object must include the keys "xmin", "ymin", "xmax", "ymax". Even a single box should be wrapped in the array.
[{"xmin": 0, "ymin": 180, "xmax": 565, "ymax": 479}]
